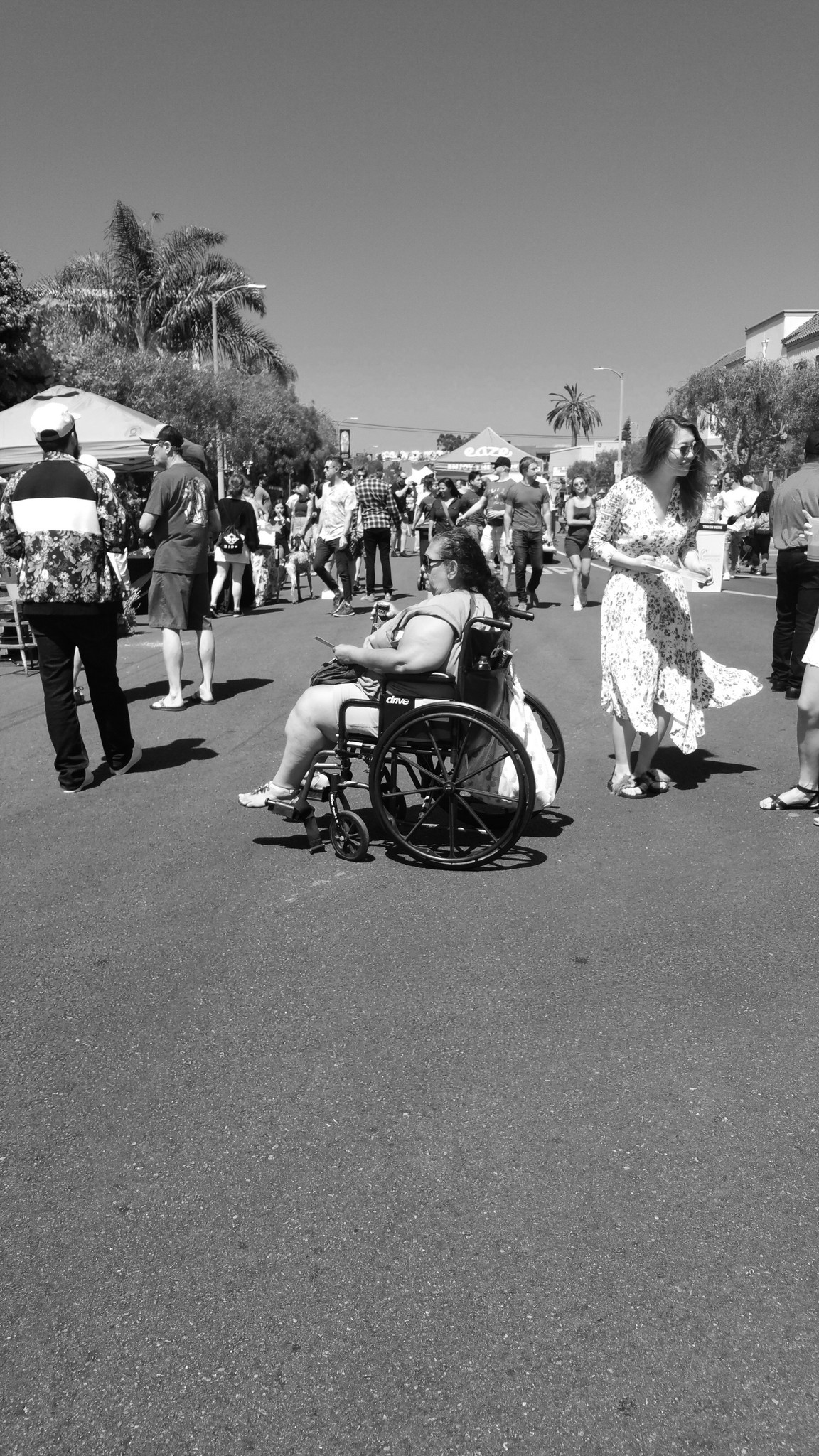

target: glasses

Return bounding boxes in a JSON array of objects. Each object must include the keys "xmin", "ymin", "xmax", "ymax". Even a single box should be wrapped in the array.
[
  {"xmin": 422, "ymin": 553, "xmax": 460, "ymax": 570},
  {"xmin": 324, "ymin": 465, "xmax": 335, "ymax": 468},
  {"xmin": 710, "ymin": 484, "xmax": 718, "ymax": 487},
  {"xmin": 666, "ymin": 440, "xmax": 703, "ymax": 457},
  {"xmin": 296, "ymin": 493, "xmax": 303, "ymax": 496},
  {"xmin": 724, "ymin": 477, "xmax": 730, "ymax": 479},
  {"xmin": 574, "ymin": 481, "xmax": 584, "ymax": 487},
  {"xmin": 357, "ymin": 475, "xmax": 367, "ymax": 478},
  {"xmin": 149, "ymin": 443, "xmax": 173, "ymax": 448},
  {"xmin": 400, "ymin": 477, "xmax": 407, "ymax": 480}
]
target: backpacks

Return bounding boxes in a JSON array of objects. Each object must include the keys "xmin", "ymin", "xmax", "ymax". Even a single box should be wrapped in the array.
[
  {"xmin": 218, "ymin": 524, "xmax": 243, "ymax": 554},
  {"xmin": 754, "ymin": 512, "xmax": 770, "ymax": 534}
]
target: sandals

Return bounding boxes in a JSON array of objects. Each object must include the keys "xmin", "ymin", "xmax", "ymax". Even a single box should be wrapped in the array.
[{"xmin": 759, "ymin": 783, "xmax": 819, "ymax": 811}]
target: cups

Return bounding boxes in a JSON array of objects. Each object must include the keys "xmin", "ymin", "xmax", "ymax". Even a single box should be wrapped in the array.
[
  {"xmin": 486, "ymin": 507, "xmax": 493, "ymax": 513},
  {"xmin": 807, "ymin": 518, "xmax": 819, "ymax": 561}
]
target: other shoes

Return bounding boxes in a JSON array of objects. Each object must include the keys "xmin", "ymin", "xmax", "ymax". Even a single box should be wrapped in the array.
[
  {"xmin": 752, "ymin": 561, "xmax": 767, "ymax": 576},
  {"xmin": 210, "ymin": 605, "xmax": 245, "ymax": 618},
  {"xmin": 391, "ymin": 551, "xmax": 411, "ymax": 557},
  {"xmin": 516, "ymin": 587, "xmax": 539, "ymax": 611},
  {"xmin": 329, "ymin": 590, "xmax": 355, "ymax": 617},
  {"xmin": 573, "ymin": 589, "xmax": 588, "ymax": 611},
  {"xmin": 722, "ymin": 569, "xmax": 736, "ymax": 580},
  {"xmin": 73, "ymin": 686, "xmax": 84, "ymax": 704},
  {"xmin": 63, "ymin": 742, "xmax": 143, "ymax": 793},
  {"xmin": 772, "ymin": 681, "xmax": 801, "ymax": 700},
  {"xmin": 237, "ymin": 770, "xmax": 331, "ymax": 808},
  {"xmin": 360, "ymin": 593, "xmax": 393, "ymax": 602}
]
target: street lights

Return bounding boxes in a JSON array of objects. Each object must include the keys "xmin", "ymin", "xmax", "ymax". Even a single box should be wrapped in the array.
[
  {"xmin": 362, "ymin": 445, "xmax": 378, "ymax": 459},
  {"xmin": 209, "ymin": 282, "xmax": 269, "ymax": 501},
  {"xmin": 592, "ymin": 367, "xmax": 624, "ymax": 484},
  {"xmin": 336, "ymin": 417, "xmax": 358, "ymax": 457}
]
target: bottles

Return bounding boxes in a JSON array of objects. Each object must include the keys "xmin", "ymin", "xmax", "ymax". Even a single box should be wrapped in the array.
[{"xmin": 473, "ymin": 656, "xmax": 491, "ymax": 670}]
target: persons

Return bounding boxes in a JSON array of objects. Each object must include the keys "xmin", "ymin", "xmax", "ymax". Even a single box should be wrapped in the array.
[
  {"xmin": 138, "ymin": 423, "xmax": 222, "ymax": 711},
  {"xmin": 770, "ymin": 430, "xmax": 819, "ymax": 700},
  {"xmin": 455, "ymin": 456, "xmax": 516, "ymax": 591},
  {"xmin": 73, "ymin": 453, "xmax": 131, "ymax": 706},
  {"xmin": 700, "ymin": 471, "xmax": 775, "ymax": 580},
  {"xmin": 503, "ymin": 457, "xmax": 554, "ymax": 611},
  {"xmin": 565, "ymin": 475, "xmax": 596, "ymax": 611},
  {"xmin": 587, "ymin": 415, "xmax": 765, "ymax": 799},
  {"xmin": 0, "ymin": 460, "xmax": 608, "ymax": 638},
  {"xmin": 0, "ymin": 404, "xmax": 143, "ymax": 792},
  {"xmin": 312, "ymin": 456, "xmax": 356, "ymax": 617},
  {"xmin": 238, "ymin": 531, "xmax": 511, "ymax": 808},
  {"xmin": 758, "ymin": 508, "xmax": 819, "ymax": 825}
]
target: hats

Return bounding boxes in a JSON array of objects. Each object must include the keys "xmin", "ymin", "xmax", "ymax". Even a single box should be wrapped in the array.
[
  {"xmin": 139, "ymin": 424, "xmax": 184, "ymax": 446},
  {"xmin": 490, "ymin": 457, "xmax": 511, "ymax": 468},
  {"xmin": 30, "ymin": 403, "xmax": 82, "ymax": 441},
  {"xmin": 257, "ymin": 473, "xmax": 271, "ymax": 479},
  {"xmin": 78, "ymin": 455, "xmax": 116, "ymax": 485},
  {"xmin": 804, "ymin": 431, "xmax": 819, "ymax": 454},
  {"xmin": 342, "ymin": 461, "xmax": 352, "ymax": 469}
]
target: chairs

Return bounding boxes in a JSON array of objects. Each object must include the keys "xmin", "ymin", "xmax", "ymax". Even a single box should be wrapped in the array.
[{"xmin": 274, "ymin": 511, "xmax": 314, "ymax": 604}]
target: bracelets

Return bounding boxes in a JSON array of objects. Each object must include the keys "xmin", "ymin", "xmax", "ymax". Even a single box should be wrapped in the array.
[
  {"xmin": 739, "ymin": 512, "xmax": 743, "ymax": 516},
  {"xmin": 411, "ymin": 527, "xmax": 415, "ymax": 531},
  {"xmin": 342, "ymin": 534, "xmax": 347, "ymax": 538},
  {"xmin": 591, "ymin": 506, "xmax": 596, "ymax": 509}
]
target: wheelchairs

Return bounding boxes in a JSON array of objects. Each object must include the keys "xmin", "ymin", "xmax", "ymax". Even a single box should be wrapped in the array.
[{"xmin": 265, "ymin": 597, "xmax": 567, "ymax": 870}]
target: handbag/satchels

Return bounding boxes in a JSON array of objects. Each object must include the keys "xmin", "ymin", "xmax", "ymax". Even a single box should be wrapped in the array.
[
  {"xmin": 310, "ymin": 659, "xmax": 368, "ymax": 687},
  {"xmin": 458, "ymin": 673, "xmax": 556, "ymax": 812}
]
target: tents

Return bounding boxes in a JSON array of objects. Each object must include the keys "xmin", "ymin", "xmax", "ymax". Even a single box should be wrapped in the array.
[
  {"xmin": 0, "ymin": 383, "xmax": 209, "ymax": 474},
  {"xmin": 434, "ymin": 426, "xmax": 544, "ymax": 479}
]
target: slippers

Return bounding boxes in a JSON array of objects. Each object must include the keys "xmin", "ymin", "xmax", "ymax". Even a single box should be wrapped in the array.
[
  {"xmin": 149, "ymin": 691, "xmax": 217, "ymax": 711},
  {"xmin": 607, "ymin": 767, "xmax": 671, "ymax": 799}
]
